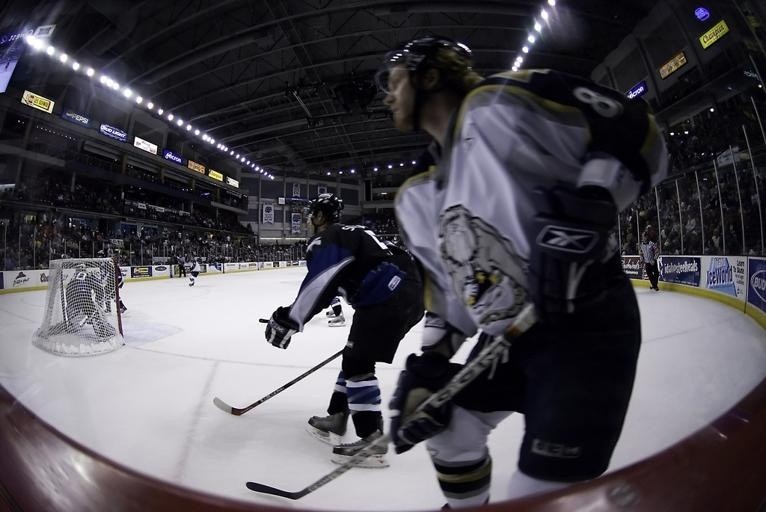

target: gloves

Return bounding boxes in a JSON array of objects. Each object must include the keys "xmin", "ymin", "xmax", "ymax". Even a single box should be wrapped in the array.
[
  {"xmin": 265, "ymin": 307, "xmax": 298, "ymax": 349},
  {"xmin": 389, "ymin": 348, "xmax": 455, "ymax": 455},
  {"xmin": 527, "ymin": 215, "xmax": 609, "ymax": 331}
]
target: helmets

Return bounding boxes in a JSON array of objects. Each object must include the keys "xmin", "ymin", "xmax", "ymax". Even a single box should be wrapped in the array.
[
  {"xmin": 373, "ymin": 35, "xmax": 472, "ymax": 97},
  {"xmin": 305, "ymin": 192, "xmax": 345, "ymax": 225}
]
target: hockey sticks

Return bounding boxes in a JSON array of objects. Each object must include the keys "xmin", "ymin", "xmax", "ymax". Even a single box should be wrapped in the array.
[
  {"xmin": 214, "ymin": 349, "xmax": 344, "ymax": 416},
  {"xmin": 245, "ymin": 233, "xmax": 620, "ymax": 500}
]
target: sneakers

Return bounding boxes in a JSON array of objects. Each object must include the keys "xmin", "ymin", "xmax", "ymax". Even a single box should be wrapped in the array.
[
  {"xmin": 307, "ymin": 412, "xmax": 348, "ymax": 438},
  {"xmin": 326, "ymin": 309, "xmax": 345, "ymax": 324},
  {"xmin": 332, "ymin": 429, "xmax": 388, "ymax": 457}
]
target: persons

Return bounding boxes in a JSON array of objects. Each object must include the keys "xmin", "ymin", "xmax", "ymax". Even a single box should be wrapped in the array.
[
  {"xmin": 186, "ymin": 254, "xmax": 201, "ymax": 288},
  {"xmin": 265, "ymin": 191, "xmax": 427, "ymax": 458},
  {"xmin": 174, "ymin": 253, "xmax": 186, "ymax": 277},
  {"xmin": 95, "ymin": 250, "xmax": 127, "ymax": 314},
  {"xmin": 639, "ymin": 231, "xmax": 660, "ymax": 291},
  {"xmin": 374, "ymin": 34, "xmax": 674, "ymax": 511},
  {"xmin": 609, "ymin": 87, "xmax": 765, "ymax": 257},
  {"xmin": 1, "ymin": 177, "xmax": 408, "ymax": 270},
  {"xmin": 38, "ymin": 263, "xmax": 117, "ymax": 340},
  {"xmin": 325, "ymin": 296, "xmax": 345, "ymax": 324}
]
